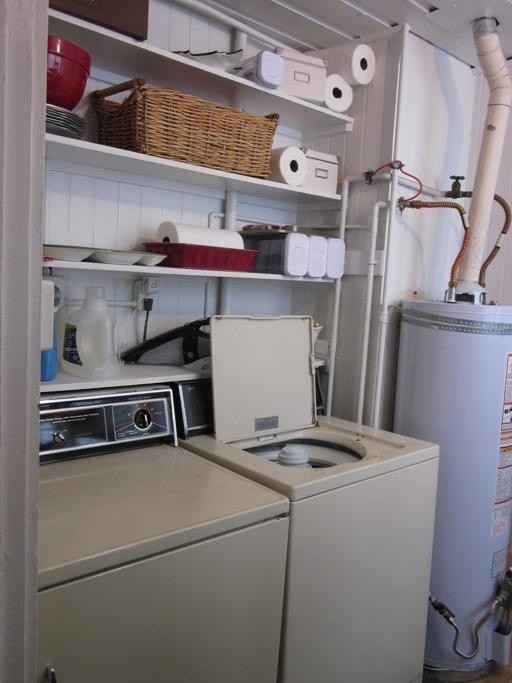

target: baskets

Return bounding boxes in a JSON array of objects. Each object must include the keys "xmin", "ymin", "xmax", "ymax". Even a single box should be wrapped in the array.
[{"xmin": 92, "ymin": 77, "xmax": 279, "ymax": 179}]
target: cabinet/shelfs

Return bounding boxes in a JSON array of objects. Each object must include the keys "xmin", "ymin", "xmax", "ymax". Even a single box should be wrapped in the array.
[{"xmin": 41, "ymin": 6, "xmax": 355, "ymax": 283}]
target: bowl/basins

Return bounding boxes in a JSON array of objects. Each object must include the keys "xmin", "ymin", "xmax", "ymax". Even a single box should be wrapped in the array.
[
  {"xmin": 41, "ymin": 242, "xmax": 96, "ymax": 261},
  {"xmin": 136, "ymin": 252, "xmax": 168, "ymax": 266},
  {"xmin": 88, "ymin": 247, "xmax": 143, "ymax": 265},
  {"xmin": 48, "ymin": 34, "xmax": 92, "ymax": 108}
]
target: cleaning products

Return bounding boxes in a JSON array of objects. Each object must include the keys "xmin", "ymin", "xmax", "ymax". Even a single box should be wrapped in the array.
[{"xmin": 59, "ymin": 285, "xmax": 123, "ymax": 381}]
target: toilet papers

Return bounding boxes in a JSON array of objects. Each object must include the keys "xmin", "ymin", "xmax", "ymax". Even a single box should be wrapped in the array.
[
  {"xmin": 154, "ymin": 220, "xmax": 245, "ymax": 250},
  {"xmin": 322, "ymin": 73, "xmax": 354, "ymax": 113},
  {"xmin": 267, "ymin": 145, "xmax": 309, "ymax": 188},
  {"xmin": 302, "ymin": 43, "xmax": 376, "ymax": 86}
]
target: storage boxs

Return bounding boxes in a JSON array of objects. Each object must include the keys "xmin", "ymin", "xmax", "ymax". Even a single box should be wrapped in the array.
[
  {"xmin": 298, "ymin": 146, "xmax": 341, "ymax": 194},
  {"xmin": 272, "ymin": 46, "xmax": 329, "ymax": 106}
]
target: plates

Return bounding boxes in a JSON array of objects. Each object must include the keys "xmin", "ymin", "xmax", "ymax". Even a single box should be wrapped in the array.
[{"xmin": 46, "ymin": 103, "xmax": 87, "ymax": 138}]
